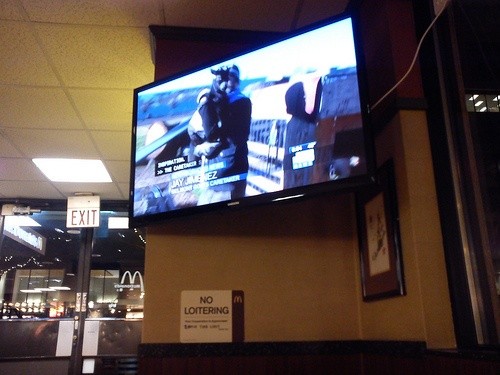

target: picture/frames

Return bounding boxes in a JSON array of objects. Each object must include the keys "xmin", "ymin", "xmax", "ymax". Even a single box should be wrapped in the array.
[{"xmin": 354, "ymin": 156, "xmax": 407, "ymax": 302}]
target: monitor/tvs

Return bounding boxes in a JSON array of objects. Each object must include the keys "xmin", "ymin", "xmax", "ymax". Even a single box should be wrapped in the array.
[{"xmin": 128, "ymin": 10, "xmax": 378, "ymax": 224}]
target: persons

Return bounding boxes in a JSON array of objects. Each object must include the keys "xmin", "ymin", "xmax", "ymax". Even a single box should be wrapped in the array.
[
  {"xmin": 192, "ymin": 65, "xmax": 252, "ymax": 206},
  {"xmin": 188, "ymin": 88, "xmax": 210, "ymax": 168}
]
